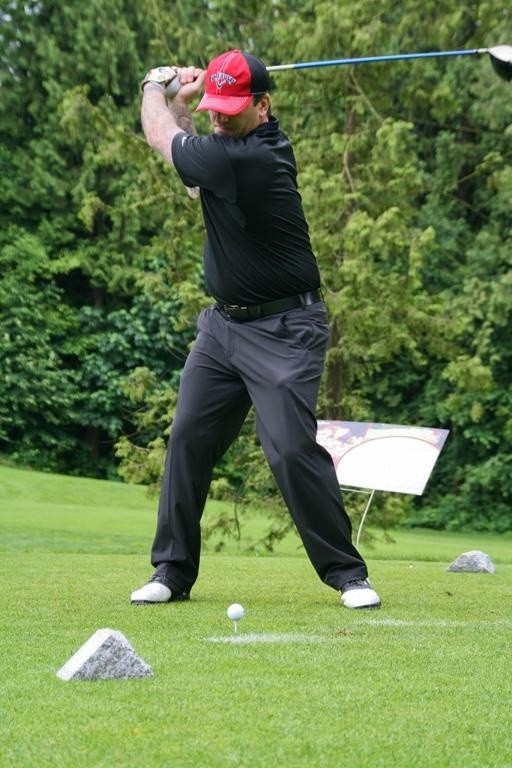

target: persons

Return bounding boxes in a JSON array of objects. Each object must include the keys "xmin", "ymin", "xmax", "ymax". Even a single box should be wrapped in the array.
[{"xmin": 127, "ymin": 45, "xmax": 384, "ymax": 612}]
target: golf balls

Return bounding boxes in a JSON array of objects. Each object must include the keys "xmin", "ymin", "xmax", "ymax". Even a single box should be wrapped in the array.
[{"xmin": 229, "ymin": 603, "xmax": 243, "ymax": 619}]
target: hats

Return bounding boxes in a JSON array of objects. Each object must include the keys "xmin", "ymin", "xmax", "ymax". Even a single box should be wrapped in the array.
[{"xmin": 195, "ymin": 48, "xmax": 271, "ymax": 117}]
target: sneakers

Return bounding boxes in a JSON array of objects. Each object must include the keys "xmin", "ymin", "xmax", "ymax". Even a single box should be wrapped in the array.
[
  {"xmin": 340, "ymin": 576, "xmax": 382, "ymax": 611},
  {"xmin": 130, "ymin": 575, "xmax": 191, "ymax": 605}
]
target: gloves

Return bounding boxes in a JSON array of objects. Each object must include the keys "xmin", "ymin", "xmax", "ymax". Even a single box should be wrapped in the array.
[{"xmin": 140, "ymin": 65, "xmax": 183, "ymax": 100}]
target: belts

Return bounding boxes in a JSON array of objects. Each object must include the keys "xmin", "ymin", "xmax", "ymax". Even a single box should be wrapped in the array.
[{"xmin": 216, "ymin": 287, "xmax": 324, "ymax": 323}]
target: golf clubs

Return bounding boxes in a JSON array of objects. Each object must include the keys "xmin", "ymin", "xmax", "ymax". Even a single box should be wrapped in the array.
[{"xmin": 193, "ymin": 45, "xmax": 511, "ymax": 82}]
